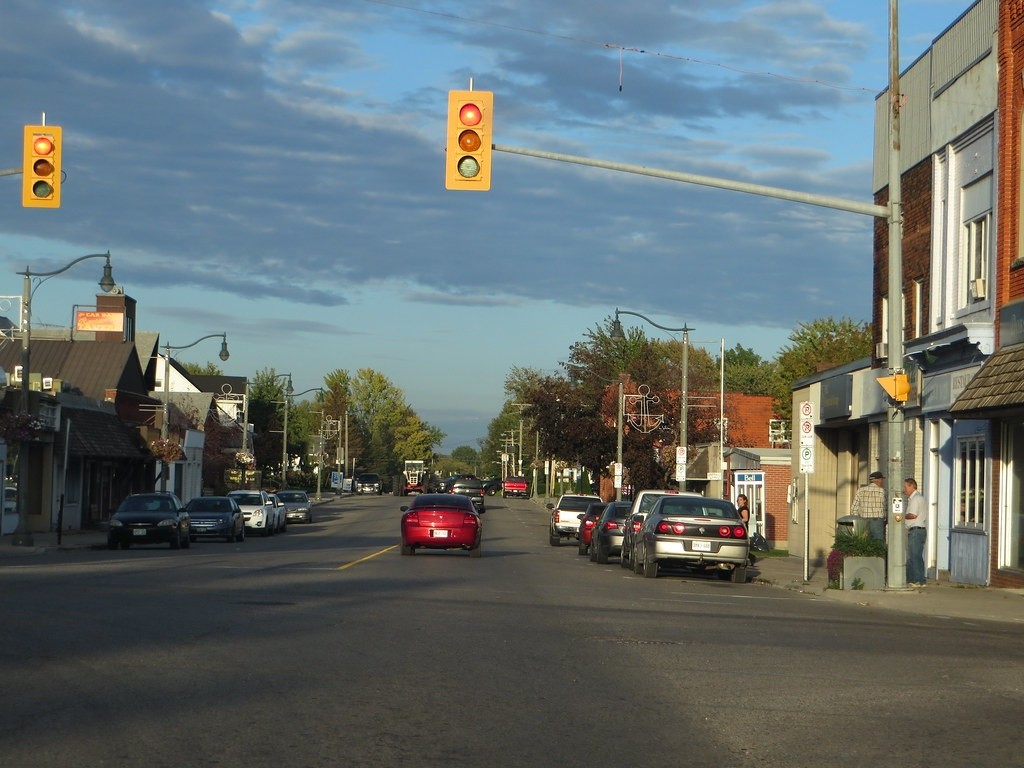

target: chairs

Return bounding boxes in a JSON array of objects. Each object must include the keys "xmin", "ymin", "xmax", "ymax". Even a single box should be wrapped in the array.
[{"xmin": 158, "ymin": 499, "xmax": 171, "ymax": 511}]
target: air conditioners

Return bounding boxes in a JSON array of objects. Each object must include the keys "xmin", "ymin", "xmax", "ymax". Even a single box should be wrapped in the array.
[
  {"xmin": 876, "ymin": 342, "xmax": 888, "ymax": 359},
  {"xmin": 970, "ymin": 279, "xmax": 986, "ymax": 298}
]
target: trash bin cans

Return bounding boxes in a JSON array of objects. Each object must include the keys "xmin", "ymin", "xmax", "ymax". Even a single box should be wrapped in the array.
[{"xmin": 837, "ymin": 516, "xmax": 865, "ymax": 537}]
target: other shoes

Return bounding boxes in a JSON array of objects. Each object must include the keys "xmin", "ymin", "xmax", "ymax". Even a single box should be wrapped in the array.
[{"xmin": 909, "ymin": 581, "xmax": 926, "ymax": 587}]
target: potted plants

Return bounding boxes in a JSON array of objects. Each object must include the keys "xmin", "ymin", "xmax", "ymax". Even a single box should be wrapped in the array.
[{"xmin": 826, "ymin": 523, "xmax": 888, "ymax": 590}]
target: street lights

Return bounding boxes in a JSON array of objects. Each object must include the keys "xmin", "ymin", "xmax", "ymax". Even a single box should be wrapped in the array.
[
  {"xmin": 160, "ymin": 331, "xmax": 230, "ymax": 494},
  {"xmin": 609, "ymin": 306, "xmax": 697, "ymax": 492},
  {"xmin": 12, "ymin": 250, "xmax": 116, "ymax": 546},
  {"xmin": 242, "ymin": 372, "xmax": 294, "ymax": 490},
  {"xmin": 573, "ymin": 365, "xmax": 624, "ymax": 502},
  {"xmin": 281, "ymin": 386, "xmax": 324, "ymax": 491}
]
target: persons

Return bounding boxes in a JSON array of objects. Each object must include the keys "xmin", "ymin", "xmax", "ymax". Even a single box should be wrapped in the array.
[
  {"xmin": 904, "ymin": 478, "xmax": 927, "ymax": 587},
  {"xmin": 737, "ymin": 494, "xmax": 753, "ymax": 568},
  {"xmin": 851, "ymin": 471, "xmax": 888, "ymax": 541}
]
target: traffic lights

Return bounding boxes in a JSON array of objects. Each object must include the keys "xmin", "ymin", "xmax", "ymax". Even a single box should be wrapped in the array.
[
  {"xmin": 444, "ymin": 90, "xmax": 494, "ymax": 193},
  {"xmin": 878, "ymin": 372, "xmax": 909, "ymax": 402},
  {"xmin": 22, "ymin": 125, "xmax": 63, "ymax": 209}
]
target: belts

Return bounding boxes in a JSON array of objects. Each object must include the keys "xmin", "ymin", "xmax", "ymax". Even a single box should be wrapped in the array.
[{"xmin": 908, "ymin": 526, "xmax": 926, "ymax": 531}]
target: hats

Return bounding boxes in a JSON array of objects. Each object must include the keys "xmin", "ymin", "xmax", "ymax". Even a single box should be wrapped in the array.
[{"xmin": 869, "ymin": 472, "xmax": 886, "ymax": 480}]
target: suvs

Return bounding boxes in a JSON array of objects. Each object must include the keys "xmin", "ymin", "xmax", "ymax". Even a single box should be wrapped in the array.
[
  {"xmin": 225, "ymin": 490, "xmax": 287, "ymax": 537},
  {"xmin": 545, "ymin": 492, "xmax": 604, "ymax": 546},
  {"xmin": 106, "ymin": 490, "xmax": 192, "ymax": 550}
]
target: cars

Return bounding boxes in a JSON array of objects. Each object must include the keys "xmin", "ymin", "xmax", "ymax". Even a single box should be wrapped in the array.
[
  {"xmin": 185, "ymin": 496, "xmax": 245, "ymax": 543},
  {"xmin": 577, "ymin": 489, "xmax": 751, "ymax": 582},
  {"xmin": 501, "ymin": 475, "xmax": 530, "ymax": 499},
  {"xmin": 357, "ymin": 473, "xmax": 384, "ymax": 495},
  {"xmin": 428, "ymin": 474, "xmax": 502, "ymax": 513},
  {"xmin": 400, "ymin": 493, "xmax": 486, "ymax": 556},
  {"xmin": 276, "ymin": 490, "xmax": 312, "ymax": 524}
]
